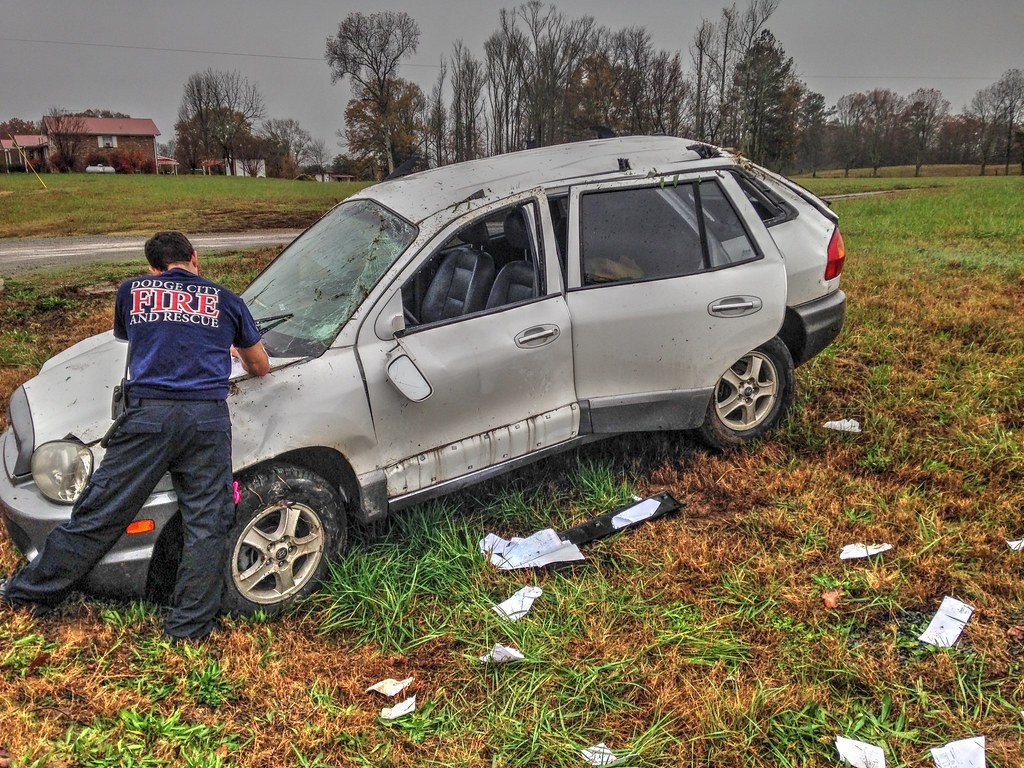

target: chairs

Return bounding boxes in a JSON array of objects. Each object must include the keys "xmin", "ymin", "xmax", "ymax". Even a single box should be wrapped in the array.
[
  {"xmin": 421, "ymin": 215, "xmax": 496, "ymax": 326},
  {"xmin": 483, "ymin": 208, "xmax": 537, "ymax": 314}
]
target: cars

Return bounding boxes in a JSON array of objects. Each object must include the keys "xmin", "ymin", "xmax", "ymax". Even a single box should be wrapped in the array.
[{"xmin": 0, "ymin": 134, "xmax": 846, "ymax": 628}]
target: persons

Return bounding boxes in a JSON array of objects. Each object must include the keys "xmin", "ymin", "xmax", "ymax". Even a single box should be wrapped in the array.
[{"xmin": 0, "ymin": 231, "xmax": 270, "ymax": 646}]
